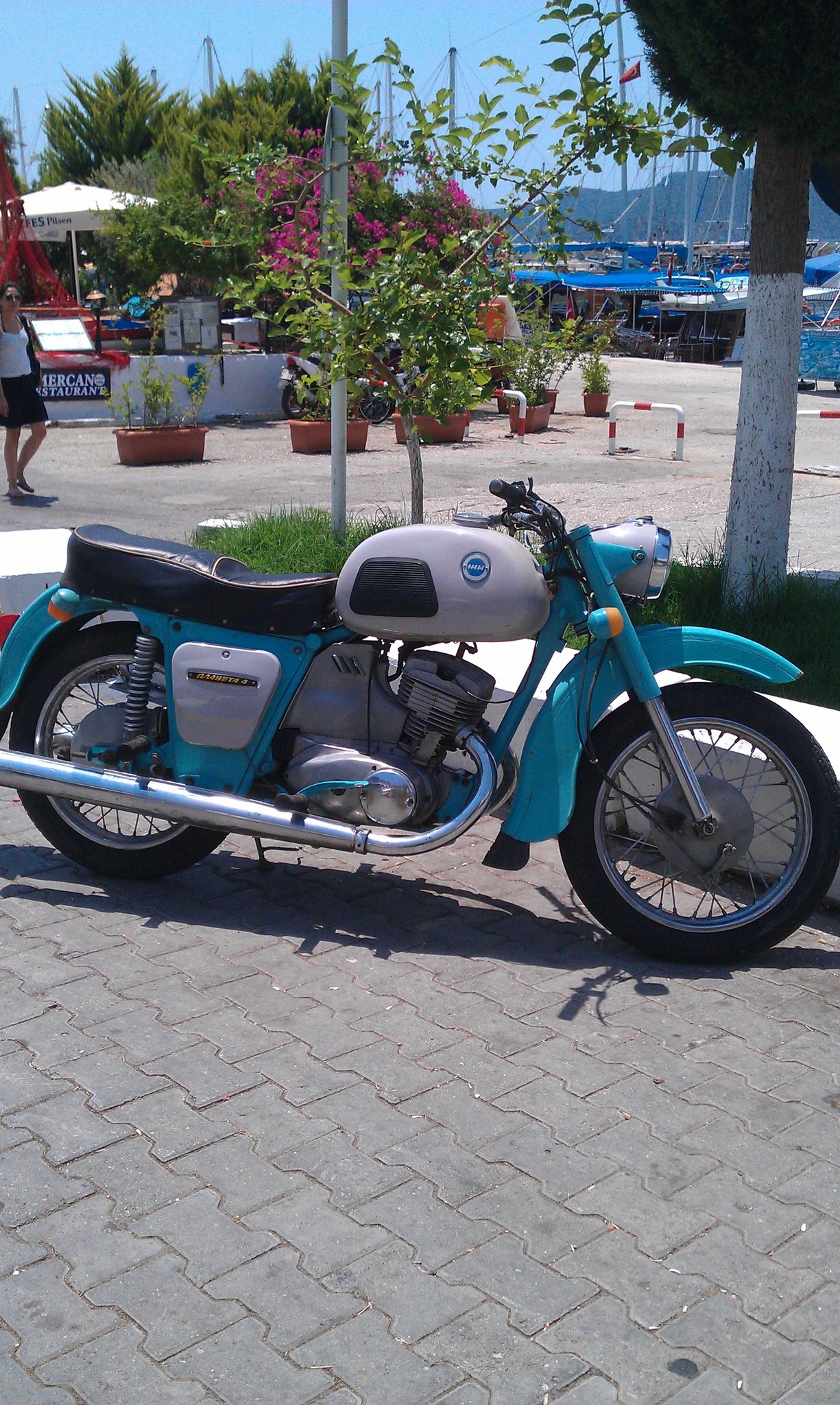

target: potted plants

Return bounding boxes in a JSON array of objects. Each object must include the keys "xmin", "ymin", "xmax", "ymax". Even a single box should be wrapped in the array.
[
  {"xmin": 284, "ymin": 307, "xmax": 632, "ymax": 455},
  {"xmin": 101, "ymin": 292, "xmax": 231, "ymax": 466}
]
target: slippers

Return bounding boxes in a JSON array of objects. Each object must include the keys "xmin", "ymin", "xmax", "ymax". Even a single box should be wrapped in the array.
[
  {"xmin": 7, "ymin": 474, "xmax": 34, "ymax": 493},
  {"xmin": 6, "ymin": 490, "xmax": 26, "ymax": 499}
]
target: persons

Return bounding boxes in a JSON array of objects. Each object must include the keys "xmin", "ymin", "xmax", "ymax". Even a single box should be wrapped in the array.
[{"xmin": 0, "ymin": 281, "xmax": 49, "ymax": 498}]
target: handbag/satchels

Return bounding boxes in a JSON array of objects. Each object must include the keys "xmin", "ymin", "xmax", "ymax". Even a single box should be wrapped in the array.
[{"xmin": 19, "ymin": 315, "xmax": 41, "ymax": 388}]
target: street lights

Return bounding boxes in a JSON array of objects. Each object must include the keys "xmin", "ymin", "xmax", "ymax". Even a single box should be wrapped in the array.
[{"xmin": 85, "ymin": 289, "xmax": 106, "ymax": 353}]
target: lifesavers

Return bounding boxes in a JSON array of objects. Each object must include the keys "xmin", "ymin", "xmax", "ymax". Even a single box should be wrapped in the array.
[
  {"xmin": 730, "ymin": 263, "xmax": 745, "ymax": 274},
  {"xmin": 721, "ymin": 268, "xmax": 730, "ymax": 275},
  {"xmin": 647, "ymin": 266, "xmax": 660, "ymax": 272},
  {"xmin": 801, "ymin": 306, "xmax": 811, "ymax": 315},
  {"xmin": 827, "ymin": 319, "xmax": 840, "ymax": 328}
]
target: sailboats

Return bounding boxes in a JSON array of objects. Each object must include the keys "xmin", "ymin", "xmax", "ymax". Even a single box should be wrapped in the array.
[{"xmin": 364, "ymin": 0, "xmax": 840, "ymax": 363}]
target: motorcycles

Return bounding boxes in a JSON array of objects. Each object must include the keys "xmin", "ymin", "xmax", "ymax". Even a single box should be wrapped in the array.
[
  {"xmin": 0, "ymin": 473, "xmax": 840, "ymax": 963},
  {"xmin": 278, "ymin": 340, "xmax": 396, "ymax": 425}
]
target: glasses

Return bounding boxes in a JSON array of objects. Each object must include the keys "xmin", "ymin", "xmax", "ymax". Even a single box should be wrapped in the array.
[{"xmin": 3, "ymin": 293, "xmax": 23, "ymax": 301}]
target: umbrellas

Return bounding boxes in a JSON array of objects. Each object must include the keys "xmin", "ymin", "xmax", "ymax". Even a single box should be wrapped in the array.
[{"xmin": 0, "ymin": 181, "xmax": 158, "ymax": 307}]
